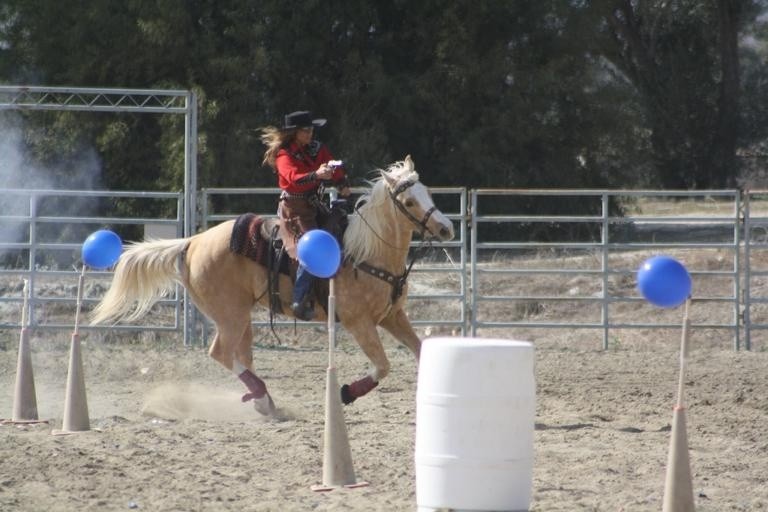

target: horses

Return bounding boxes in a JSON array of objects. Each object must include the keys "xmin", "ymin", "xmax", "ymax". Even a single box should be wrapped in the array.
[{"xmin": 87, "ymin": 153, "xmax": 457, "ymax": 420}]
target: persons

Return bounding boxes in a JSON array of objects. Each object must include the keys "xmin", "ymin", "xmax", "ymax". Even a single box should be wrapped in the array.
[{"xmin": 253, "ymin": 107, "xmax": 354, "ymax": 319}]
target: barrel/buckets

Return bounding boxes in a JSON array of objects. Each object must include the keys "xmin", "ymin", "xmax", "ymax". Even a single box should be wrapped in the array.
[{"xmin": 415, "ymin": 335, "xmax": 536, "ymax": 511}]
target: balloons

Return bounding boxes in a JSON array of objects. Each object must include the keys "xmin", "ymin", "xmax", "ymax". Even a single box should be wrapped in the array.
[
  {"xmin": 294, "ymin": 227, "xmax": 340, "ymax": 279},
  {"xmin": 81, "ymin": 229, "xmax": 123, "ymax": 270},
  {"xmin": 635, "ymin": 256, "xmax": 691, "ymax": 309}
]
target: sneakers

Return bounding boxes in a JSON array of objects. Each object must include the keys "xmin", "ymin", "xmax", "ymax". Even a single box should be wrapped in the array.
[{"xmin": 290, "ymin": 302, "xmax": 314, "ymax": 321}]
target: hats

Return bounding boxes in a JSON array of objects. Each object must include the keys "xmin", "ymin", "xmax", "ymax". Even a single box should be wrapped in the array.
[{"xmin": 283, "ymin": 111, "xmax": 327, "ymax": 129}]
